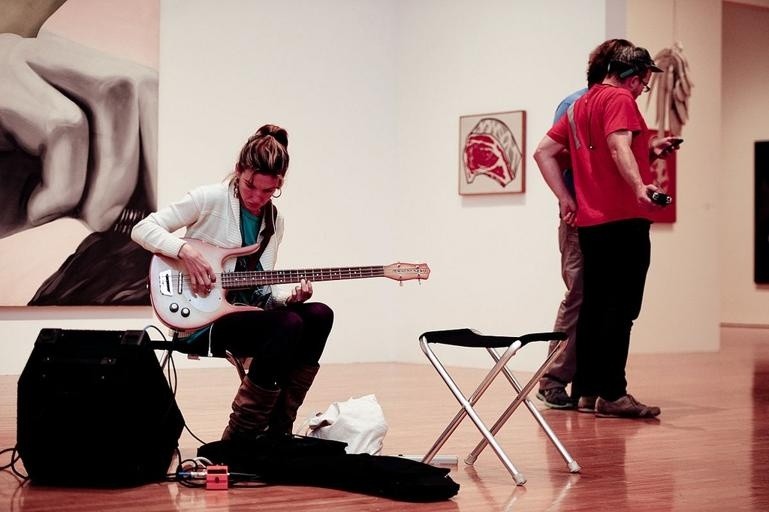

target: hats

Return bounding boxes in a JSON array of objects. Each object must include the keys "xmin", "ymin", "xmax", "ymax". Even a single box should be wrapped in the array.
[{"xmin": 613, "ymin": 46, "xmax": 664, "ymax": 74}]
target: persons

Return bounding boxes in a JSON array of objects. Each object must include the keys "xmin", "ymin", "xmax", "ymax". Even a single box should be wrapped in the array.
[
  {"xmin": 0, "ymin": 2, "xmax": 159, "ymax": 232},
  {"xmin": 131, "ymin": 125, "xmax": 333, "ymax": 442},
  {"xmin": 535, "ymin": 38, "xmax": 684, "ymax": 408},
  {"xmin": 533, "ymin": 47, "xmax": 666, "ymax": 418}
]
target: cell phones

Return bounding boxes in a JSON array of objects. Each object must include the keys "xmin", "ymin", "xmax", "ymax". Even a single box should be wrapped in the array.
[{"xmin": 660, "ymin": 139, "xmax": 684, "ymax": 155}]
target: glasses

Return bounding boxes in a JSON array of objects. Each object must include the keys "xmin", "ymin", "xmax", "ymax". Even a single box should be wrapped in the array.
[{"xmin": 641, "ymin": 80, "xmax": 652, "ymax": 94}]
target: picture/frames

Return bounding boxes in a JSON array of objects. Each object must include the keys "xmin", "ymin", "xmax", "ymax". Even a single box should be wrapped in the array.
[{"xmin": 457, "ymin": 110, "xmax": 528, "ymax": 196}]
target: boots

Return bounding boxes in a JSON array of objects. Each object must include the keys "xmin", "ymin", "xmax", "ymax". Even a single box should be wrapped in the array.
[
  {"xmin": 220, "ymin": 375, "xmax": 280, "ymax": 443},
  {"xmin": 266, "ymin": 361, "xmax": 321, "ymax": 436}
]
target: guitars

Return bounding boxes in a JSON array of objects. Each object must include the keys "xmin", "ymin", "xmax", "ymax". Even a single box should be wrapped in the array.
[{"xmin": 148, "ymin": 237, "xmax": 430, "ymax": 334}]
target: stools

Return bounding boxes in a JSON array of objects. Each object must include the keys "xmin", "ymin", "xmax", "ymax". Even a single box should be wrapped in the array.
[
  {"xmin": 414, "ymin": 328, "xmax": 581, "ymax": 485},
  {"xmin": 144, "ymin": 338, "xmax": 245, "ymax": 401}
]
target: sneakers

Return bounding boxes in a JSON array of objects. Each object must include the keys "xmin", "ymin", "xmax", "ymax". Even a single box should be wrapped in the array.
[
  {"xmin": 536, "ymin": 387, "xmax": 572, "ymax": 409},
  {"xmin": 595, "ymin": 394, "xmax": 661, "ymax": 418},
  {"xmin": 578, "ymin": 396, "xmax": 595, "ymax": 412}
]
